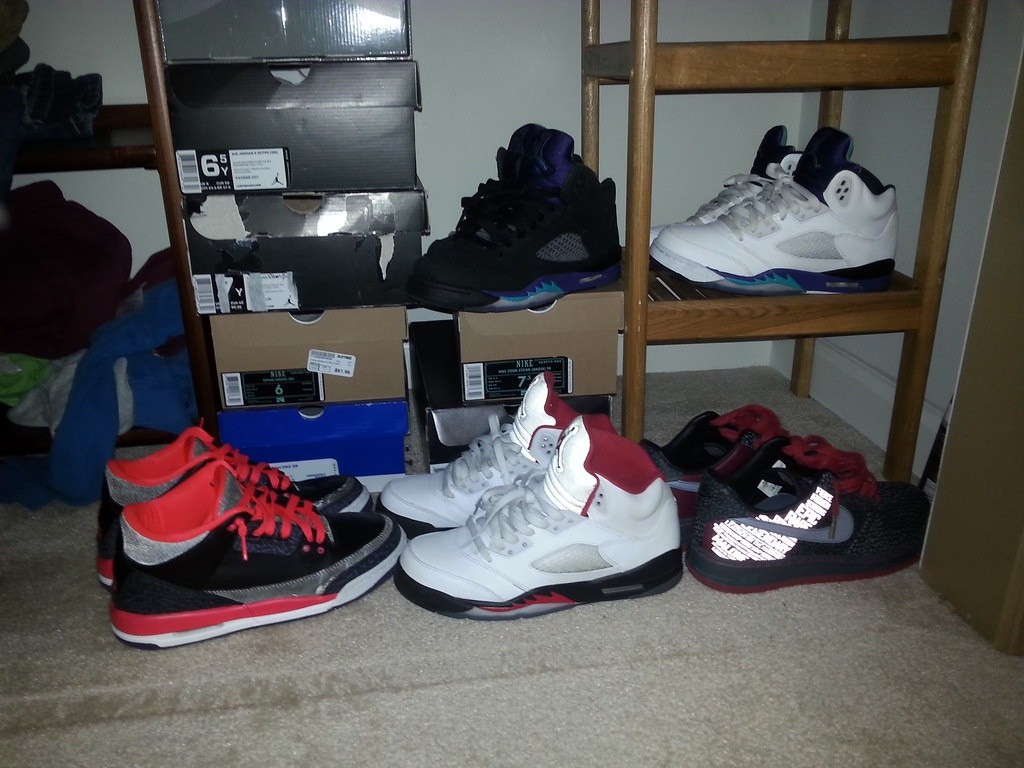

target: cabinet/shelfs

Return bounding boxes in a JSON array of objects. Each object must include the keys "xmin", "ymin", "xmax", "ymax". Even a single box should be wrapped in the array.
[
  {"xmin": 0, "ymin": 0, "xmax": 239, "ymax": 486},
  {"xmin": 577, "ymin": 0, "xmax": 986, "ymax": 534}
]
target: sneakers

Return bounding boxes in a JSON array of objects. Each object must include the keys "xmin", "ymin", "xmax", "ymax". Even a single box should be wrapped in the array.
[
  {"xmin": 687, "ymin": 430, "xmax": 930, "ymax": 594},
  {"xmin": 107, "ymin": 459, "xmax": 409, "ymax": 649},
  {"xmin": 374, "ymin": 373, "xmax": 619, "ymax": 540},
  {"xmin": 650, "ymin": 125, "xmax": 898, "ymax": 291},
  {"xmin": 639, "ymin": 401, "xmax": 796, "ymax": 552},
  {"xmin": 96, "ymin": 419, "xmax": 369, "ymax": 585},
  {"xmin": 396, "ymin": 416, "xmax": 683, "ymax": 621},
  {"xmin": 408, "ymin": 124, "xmax": 621, "ymax": 311}
]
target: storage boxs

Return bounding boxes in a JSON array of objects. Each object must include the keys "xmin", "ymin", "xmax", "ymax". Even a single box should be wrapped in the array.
[{"xmin": 155, "ymin": 0, "xmax": 624, "ymax": 495}]
